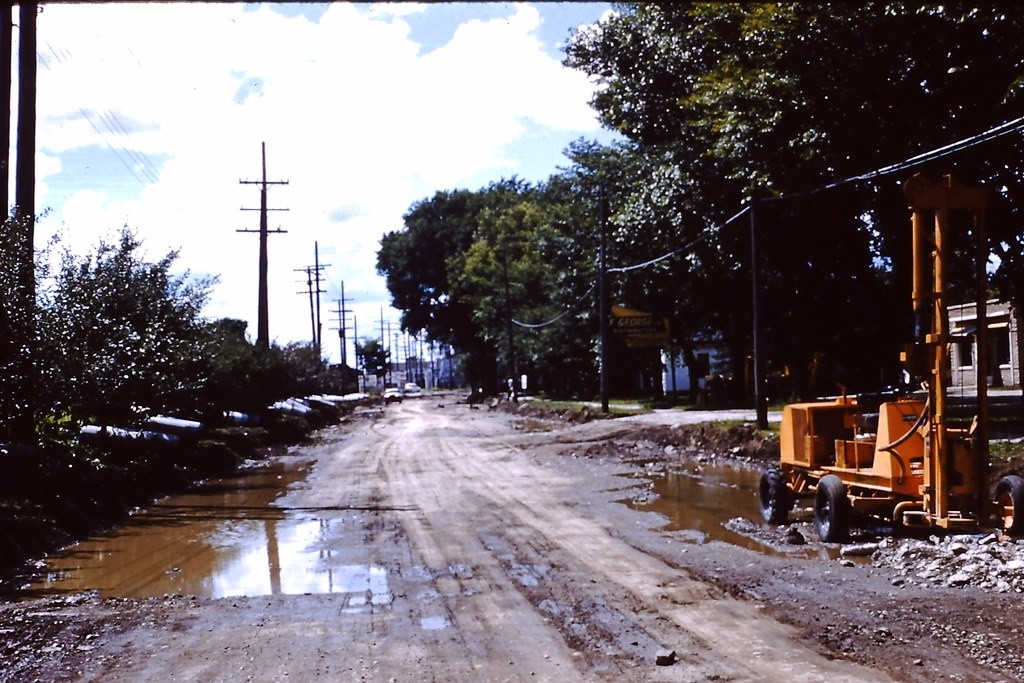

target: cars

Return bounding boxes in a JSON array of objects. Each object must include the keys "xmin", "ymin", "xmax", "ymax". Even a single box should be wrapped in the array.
[
  {"xmin": 404, "ymin": 383, "xmax": 424, "ymax": 400},
  {"xmin": 383, "ymin": 387, "xmax": 403, "ymax": 403}
]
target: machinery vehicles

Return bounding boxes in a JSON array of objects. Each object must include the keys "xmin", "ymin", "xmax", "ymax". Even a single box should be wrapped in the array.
[
  {"xmin": 757, "ymin": 185, "xmax": 1024, "ymax": 553},
  {"xmin": 611, "ymin": 302, "xmax": 826, "ymax": 412}
]
